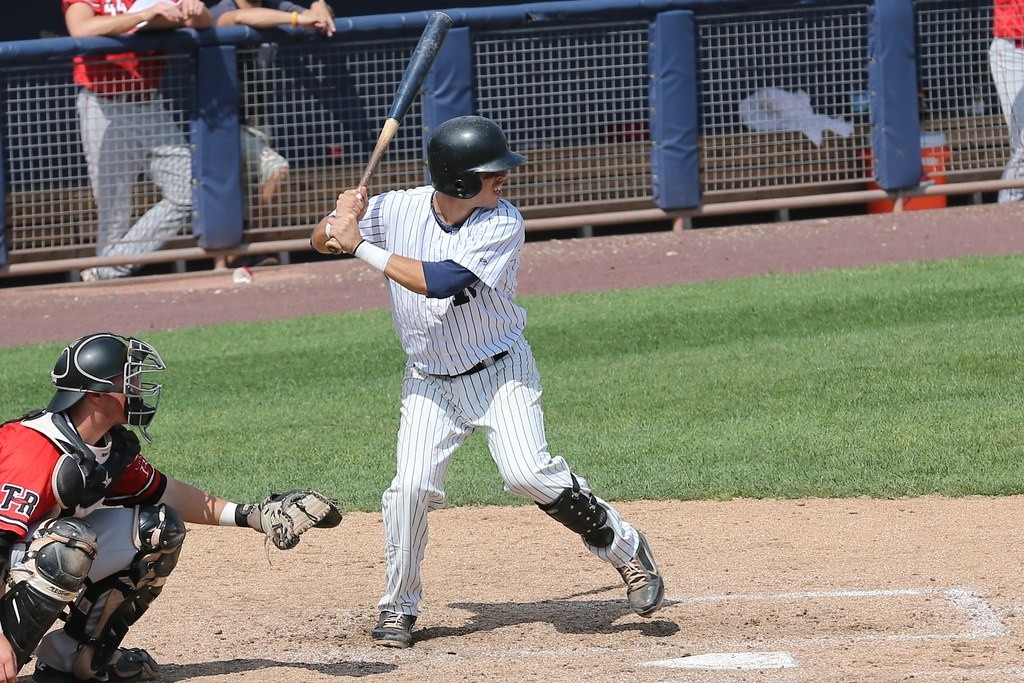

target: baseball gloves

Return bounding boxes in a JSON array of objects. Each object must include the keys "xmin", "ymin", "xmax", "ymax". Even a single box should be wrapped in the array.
[{"xmin": 235, "ymin": 489, "xmax": 343, "ymax": 551}]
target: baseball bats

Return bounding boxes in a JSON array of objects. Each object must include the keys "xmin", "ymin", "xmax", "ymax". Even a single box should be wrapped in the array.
[{"xmin": 325, "ymin": 11, "xmax": 453, "ymax": 257}]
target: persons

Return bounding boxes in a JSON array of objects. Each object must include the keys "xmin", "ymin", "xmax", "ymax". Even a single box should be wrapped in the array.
[
  {"xmin": 62, "ymin": 1, "xmax": 338, "ymax": 283},
  {"xmin": 309, "ymin": 114, "xmax": 665, "ymax": 651},
  {"xmin": 988, "ymin": 0, "xmax": 1024, "ymax": 204},
  {"xmin": 0, "ymin": 331, "xmax": 343, "ymax": 682}
]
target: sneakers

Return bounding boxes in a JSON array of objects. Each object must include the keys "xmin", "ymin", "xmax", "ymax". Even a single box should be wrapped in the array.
[
  {"xmin": 616, "ymin": 530, "xmax": 666, "ymax": 616},
  {"xmin": 372, "ymin": 610, "xmax": 417, "ymax": 647}
]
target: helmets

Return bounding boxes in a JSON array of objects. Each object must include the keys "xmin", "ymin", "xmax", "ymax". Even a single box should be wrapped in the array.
[
  {"xmin": 426, "ymin": 115, "xmax": 527, "ymax": 198},
  {"xmin": 45, "ymin": 330, "xmax": 157, "ymax": 426}
]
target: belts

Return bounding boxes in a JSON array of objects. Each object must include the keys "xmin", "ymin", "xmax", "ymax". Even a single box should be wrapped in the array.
[{"xmin": 443, "ymin": 351, "xmax": 508, "ymax": 378}]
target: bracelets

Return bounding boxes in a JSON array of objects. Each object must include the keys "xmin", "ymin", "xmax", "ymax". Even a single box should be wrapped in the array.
[
  {"xmin": 352, "ymin": 239, "xmax": 392, "ymax": 273},
  {"xmin": 291, "ymin": 11, "xmax": 299, "ymax": 27},
  {"xmin": 218, "ymin": 501, "xmax": 238, "ymax": 527}
]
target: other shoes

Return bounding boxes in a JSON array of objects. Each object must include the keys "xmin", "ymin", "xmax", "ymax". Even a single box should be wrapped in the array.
[
  {"xmin": 32, "ymin": 645, "xmax": 158, "ymax": 683},
  {"xmin": 80, "ymin": 267, "xmax": 98, "ymax": 282}
]
